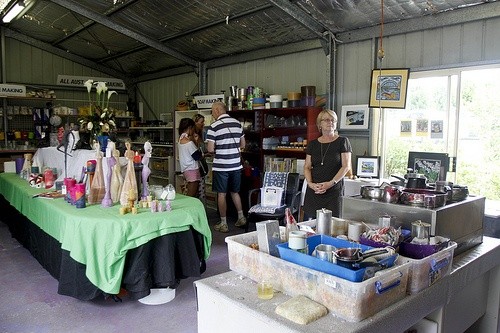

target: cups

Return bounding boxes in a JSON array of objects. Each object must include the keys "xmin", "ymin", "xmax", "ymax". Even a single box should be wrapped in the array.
[
  {"xmin": 412, "ymin": 221, "xmax": 432, "ymax": 239},
  {"xmin": 257, "ymin": 275, "xmax": 274, "ymax": 300},
  {"xmin": 289, "ymin": 209, "xmax": 367, "ymax": 264},
  {"xmin": 223, "ymin": 84, "xmax": 315, "ymax": 111}
]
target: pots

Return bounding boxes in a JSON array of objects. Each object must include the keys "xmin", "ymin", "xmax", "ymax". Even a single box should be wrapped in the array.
[
  {"xmin": 333, "ymin": 243, "xmax": 388, "ymax": 269},
  {"xmin": 360, "ymin": 174, "xmax": 470, "ymax": 208}
]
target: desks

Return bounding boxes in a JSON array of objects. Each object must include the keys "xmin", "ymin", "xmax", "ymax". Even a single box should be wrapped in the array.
[
  {"xmin": 194, "ymin": 235, "xmax": 500, "ymax": 333},
  {"xmin": 0, "ymin": 172, "xmax": 212, "ymax": 306}
]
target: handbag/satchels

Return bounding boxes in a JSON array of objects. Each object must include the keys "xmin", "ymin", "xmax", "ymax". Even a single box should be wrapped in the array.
[{"xmin": 242, "ymin": 160, "xmax": 252, "ymax": 176}]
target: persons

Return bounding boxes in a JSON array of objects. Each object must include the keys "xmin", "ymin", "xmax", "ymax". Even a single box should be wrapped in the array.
[
  {"xmin": 207, "ymin": 102, "xmax": 246, "ymax": 233},
  {"xmin": 303, "ymin": 109, "xmax": 352, "ymax": 221},
  {"xmin": 182, "ymin": 114, "xmax": 207, "ymax": 204},
  {"xmin": 179, "ymin": 118, "xmax": 204, "ymax": 199}
]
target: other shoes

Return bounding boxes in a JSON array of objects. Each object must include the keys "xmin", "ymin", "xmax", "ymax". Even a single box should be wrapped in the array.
[
  {"xmin": 234, "ymin": 217, "xmax": 246, "ymax": 226},
  {"xmin": 214, "ymin": 223, "xmax": 229, "ymax": 233}
]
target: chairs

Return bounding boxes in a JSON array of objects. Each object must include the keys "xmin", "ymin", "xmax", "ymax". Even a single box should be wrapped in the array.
[{"xmin": 249, "ymin": 171, "xmax": 303, "ymax": 233}]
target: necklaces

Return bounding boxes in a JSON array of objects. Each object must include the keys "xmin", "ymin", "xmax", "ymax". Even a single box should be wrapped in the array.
[{"xmin": 321, "ymin": 142, "xmax": 331, "ymax": 165}]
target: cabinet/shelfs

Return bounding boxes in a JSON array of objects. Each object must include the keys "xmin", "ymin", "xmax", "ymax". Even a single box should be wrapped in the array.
[{"xmin": 0, "ymin": 96, "xmax": 325, "ymax": 212}]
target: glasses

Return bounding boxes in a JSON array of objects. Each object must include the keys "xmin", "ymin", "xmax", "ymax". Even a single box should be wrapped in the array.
[{"xmin": 320, "ymin": 118, "xmax": 337, "ymax": 123}]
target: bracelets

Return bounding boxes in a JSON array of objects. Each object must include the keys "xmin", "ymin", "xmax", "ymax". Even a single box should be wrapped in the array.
[{"xmin": 333, "ymin": 179, "xmax": 337, "ymax": 184}]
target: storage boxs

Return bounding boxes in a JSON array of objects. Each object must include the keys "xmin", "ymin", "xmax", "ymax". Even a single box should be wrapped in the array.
[{"xmin": 225, "ymin": 216, "xmax": 457, "ymax": 323}]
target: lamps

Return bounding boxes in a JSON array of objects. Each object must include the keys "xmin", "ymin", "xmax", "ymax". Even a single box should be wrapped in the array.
[{"xmin": 1, "ymin": 0, "xmax": 25, "ymax": 23}]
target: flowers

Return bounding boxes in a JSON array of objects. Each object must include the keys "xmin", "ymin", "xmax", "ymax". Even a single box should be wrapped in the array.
[
  {"xmin": 76, "ymin": 79, "xmax": 118, "ymax": 147},
  {"xmin": 417, "ymin": 160, "xmax": 441, "ymax": 183}
]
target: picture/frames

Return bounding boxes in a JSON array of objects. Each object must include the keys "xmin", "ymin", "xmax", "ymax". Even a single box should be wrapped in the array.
[
  {"xmin": 368, "ymin": 68, "xmax": 411, "ymax": 109},
  {"xmin": 355, "ymin": 155, "xmax": 381, "ymax": 179},
  {"xmin": 339, "ymin": 104, "xmax": 369, "ymax": 129},
  {"xmin": 408, "ymin": 152, "xmax": 448, "ymax": 184}
]
